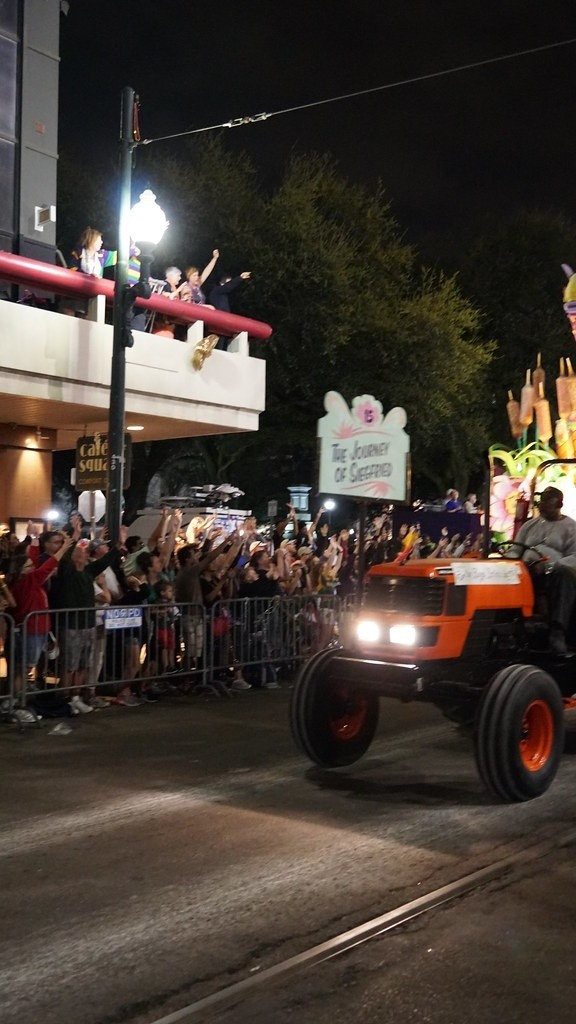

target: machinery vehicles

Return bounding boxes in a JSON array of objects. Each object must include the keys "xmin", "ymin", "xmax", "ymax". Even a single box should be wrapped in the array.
[{"xmin": 289, "ymin": 458, "xmax": 576, "ymax": 804}]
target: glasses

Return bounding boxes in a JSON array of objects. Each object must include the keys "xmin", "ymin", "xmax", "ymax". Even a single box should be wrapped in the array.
[{"xmin": 139, "ymin": 541, "xmax": 145, "ymax": 546}]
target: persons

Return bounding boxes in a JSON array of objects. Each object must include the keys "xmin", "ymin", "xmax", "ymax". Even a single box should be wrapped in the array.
[
  {"xmin": 0, "ymin": 489, "xmax": 484, "ymax": 722},
  {"xmin": 505, "ymin": 486, "xmax": 576, "ymax": 652},
  {"xmin": 62, "ymin": 227, "xmax": 251, "ymax": 350}
]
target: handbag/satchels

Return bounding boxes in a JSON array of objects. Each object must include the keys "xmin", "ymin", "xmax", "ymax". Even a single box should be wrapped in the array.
[
  {"xmin": 42, "ymin": 631, "xmax": 61, "ymax": 660},
  {"xmin": 207, "ymin": 606, "xmax": 232, "ymax": 637}
]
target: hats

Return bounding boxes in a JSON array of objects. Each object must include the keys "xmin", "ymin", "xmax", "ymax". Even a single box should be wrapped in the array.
[
  {"xmin": 87, "ymin": 537, "xmax": 111, "ymax": 549},
  {"xmin": 532, "ymin": 486, "xmax": 564, "ymax": 499},
  {"xmin": 298, "ymin": 521, "xmax": 307, "ymax": 529},
  {"xmin": 248, "ymin": 541, "xmax": 268, "ymax": 552},
  {"xmin": 297, "ymin": 546, "xmax": 314, "ymax": 556},
  {"xmin": 77, "ymin": 538, "xmax": 90, "ymax": 549}
]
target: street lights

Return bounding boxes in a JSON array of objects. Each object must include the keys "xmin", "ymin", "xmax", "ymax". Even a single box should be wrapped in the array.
[
  {"xmin": 107, "ymin": 89, "xmax": 170, "ymax": 540},
  {"xmin": 325, "ymin": 499, "xmax": 336, "ymax": 526}
]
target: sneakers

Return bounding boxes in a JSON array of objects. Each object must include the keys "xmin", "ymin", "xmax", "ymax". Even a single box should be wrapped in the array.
[
  {"xmin": 0, "ymin": 698, "xmax": 19, "ymax": 713},
  {"xmin": 71, "ymin": 695, "xmax": 94, "ymax": 715},
  {"xmin": 140, "ymin": 687, "xmax": 161, "ymax": 702},
  {"xmin": 68, "ymin": 701, "xmax": 79, "ymax": 715},
  {"xmin": 227, "ymin": 677, "xmax": 252, "ymax": 690},
  {"xmin": 263, "ymin": 682, "xmax": 281, "ymax": 688},
  {"xmin": 11, "ymin": 709, "xmax": 42, "ymax": 722},
  {"xmin": 86, "ymin": 695, "xmax": 111, "ymax": 708},
  {"xmin": 115, "ymin": 693, "xmax": 141, "ymax": 706}
]
